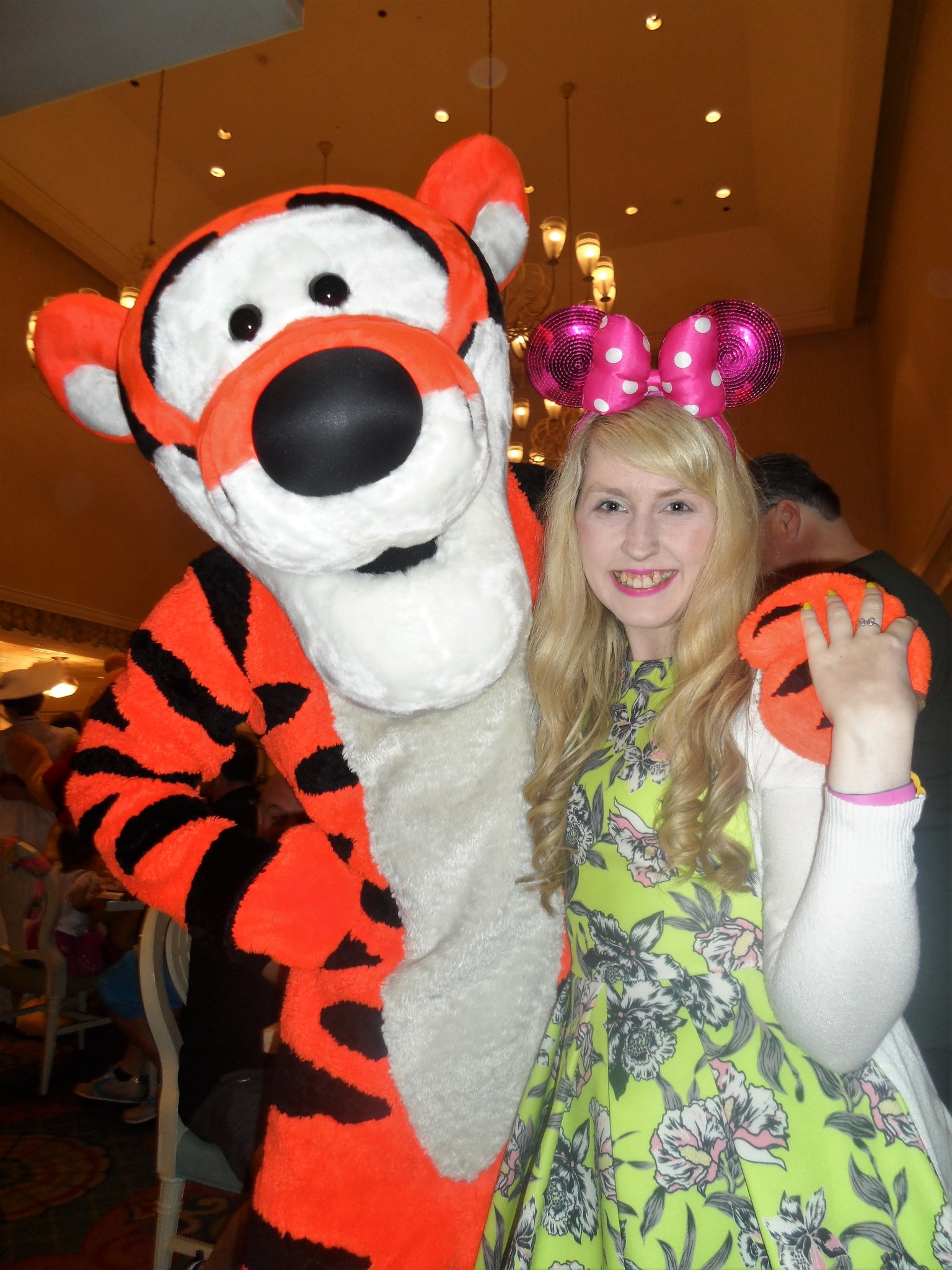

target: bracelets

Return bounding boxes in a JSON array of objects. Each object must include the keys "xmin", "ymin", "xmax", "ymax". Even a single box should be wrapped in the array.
[{"xmin": 827, "ymin": 770, "xmax": 923, "ymax": 808}]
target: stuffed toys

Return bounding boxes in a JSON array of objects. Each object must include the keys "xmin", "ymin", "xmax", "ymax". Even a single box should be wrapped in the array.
[{"xmin": 28, "ymin": 131, "xmax": 952, "ymax": 1270}]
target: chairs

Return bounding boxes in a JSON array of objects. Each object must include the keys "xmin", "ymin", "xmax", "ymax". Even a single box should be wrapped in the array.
[
  {"xmin": 138, "ymin": 898, "xmax": 252, "ymax": 1269},
  {"xmin": 0, "ymin": 833, "xmax": 113, "ymax": 1098}
]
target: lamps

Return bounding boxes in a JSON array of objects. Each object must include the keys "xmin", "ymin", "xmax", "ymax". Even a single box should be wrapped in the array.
[
  {"xmin": 509, "ymin": 384, "xmax": 565, "ymax": 462},
  {"xmin": 536, "ymin": 212, "xmax": 619, "ymax": 314}
]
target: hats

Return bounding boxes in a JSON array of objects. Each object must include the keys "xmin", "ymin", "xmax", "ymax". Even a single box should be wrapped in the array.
[{"xmin": 0, "ymin": 732, "xmax": 57, "ymax": 812}]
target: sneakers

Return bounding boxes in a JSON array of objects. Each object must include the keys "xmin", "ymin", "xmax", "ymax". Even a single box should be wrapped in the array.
[
  {"xmin": 119, "ymin": 1081, "xmax": 162, "ymax": 1124},
  {"xmin": 73, "ymin": 1073, "xmax": 145, "ymax": 1104}
]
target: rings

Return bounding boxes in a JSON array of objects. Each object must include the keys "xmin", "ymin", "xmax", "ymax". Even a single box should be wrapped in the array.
[{"xmin": 856, "ymin": 616, "xmax": 883, "ymax": 632}]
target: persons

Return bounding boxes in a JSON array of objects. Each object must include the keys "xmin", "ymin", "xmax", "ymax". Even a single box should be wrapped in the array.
[
  {"xmin": 0, "ymin": 663, "xmax": 317, "ymax": 1270},
  {"xmin": 747, "ymin": 447, "xmax": 952, "ymax": 1111},
  {"xmin": 470, "ymin": 310, "xmax": 952, "ymax": 1270}
]
